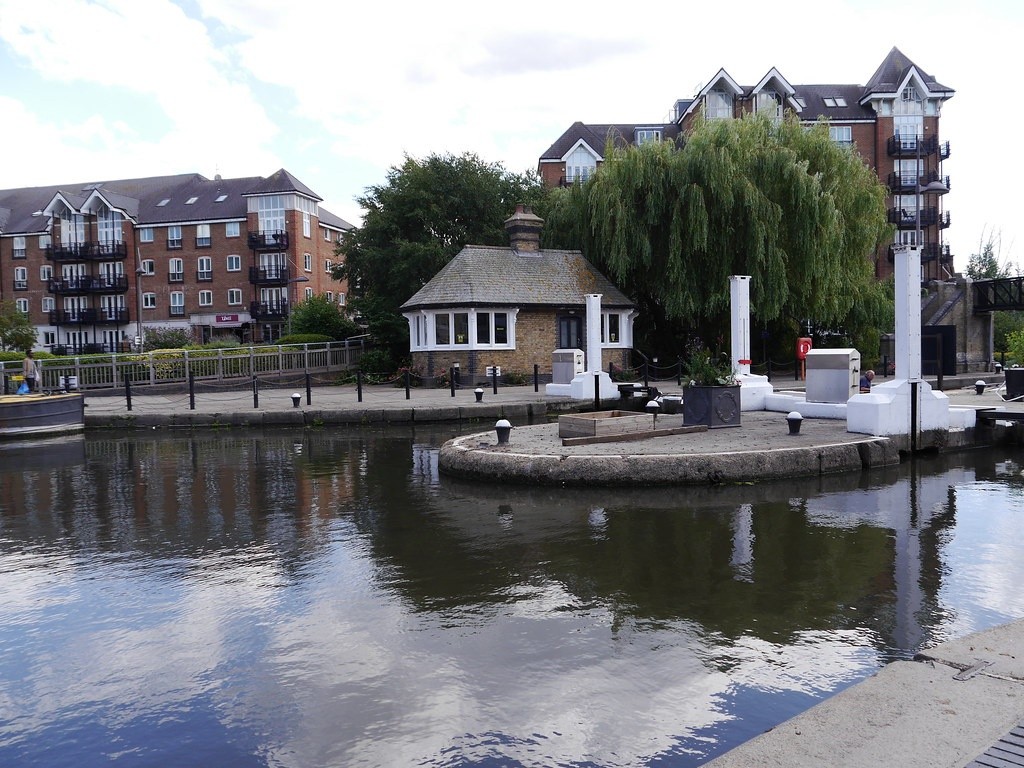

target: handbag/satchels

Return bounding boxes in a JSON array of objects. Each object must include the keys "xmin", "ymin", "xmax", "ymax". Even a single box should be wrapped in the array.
[
  {"xmin": 16, "ymin": 383, "xmax": 30, "ymax": 394},
  {"xmin": 33, "ymin": 369, "xmax": 41, "ymax": 382}
]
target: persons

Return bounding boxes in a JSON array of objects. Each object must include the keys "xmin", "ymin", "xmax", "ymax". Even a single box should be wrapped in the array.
[
  {"xmin": 22, "ymin": 350, "xmax": 39, "ymax": 392},
  {"xmin": 860, "ymin": 370, "xmax": 875, "ymax": 393}
]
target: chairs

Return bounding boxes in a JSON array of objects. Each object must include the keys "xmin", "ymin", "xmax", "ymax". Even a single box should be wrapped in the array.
[{"xmin": 902, "ymin": 209, "xmax": 914, "ymax": 221}]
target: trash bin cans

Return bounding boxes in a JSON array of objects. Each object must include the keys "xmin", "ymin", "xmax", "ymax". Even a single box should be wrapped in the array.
[{"xmin": 1003, "ymin": 368, "xmax": 1024, "ymax": 398}]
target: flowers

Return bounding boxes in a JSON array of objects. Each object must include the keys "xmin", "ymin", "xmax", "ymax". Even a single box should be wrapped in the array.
[{"xmin": 677, "ymin": 333, "xmax": 741, "ymax": 387}]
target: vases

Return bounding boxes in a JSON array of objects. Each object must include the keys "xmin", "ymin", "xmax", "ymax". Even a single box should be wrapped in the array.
[{"xmin": 683, "ymin": 386, "xmax": 741, "ymax": 428}]
